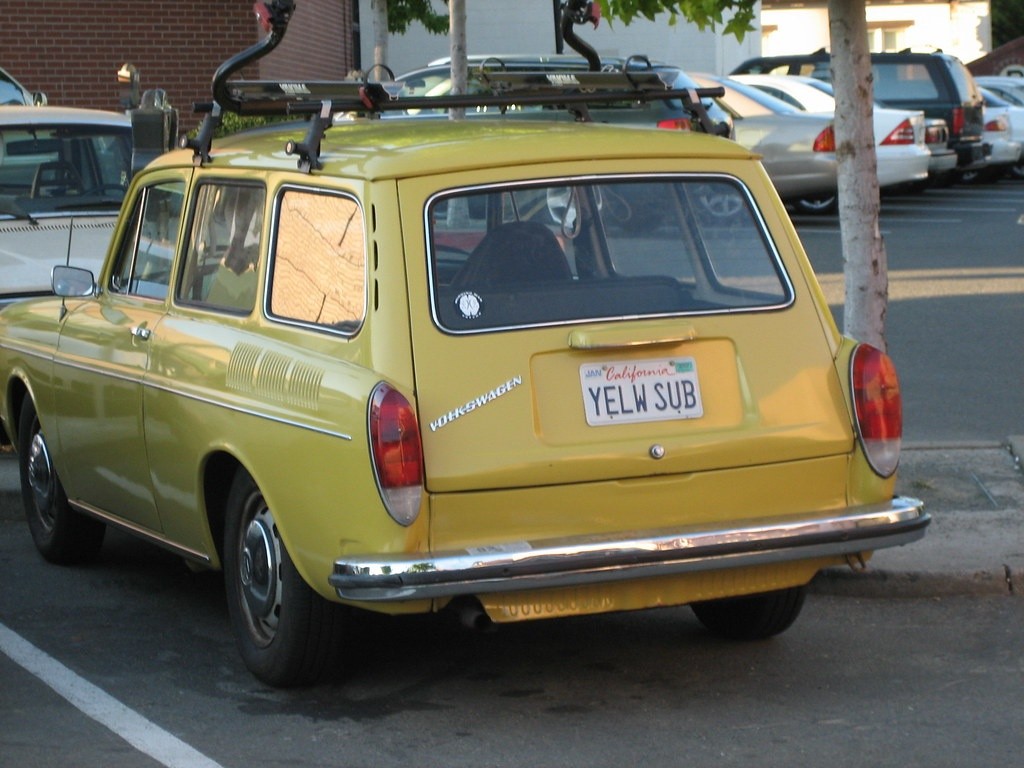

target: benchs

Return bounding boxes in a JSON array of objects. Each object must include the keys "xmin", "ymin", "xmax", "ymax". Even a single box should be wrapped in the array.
[{"xmin": 426, "ymin": 276, "xmax": 693, "ymax": 336}]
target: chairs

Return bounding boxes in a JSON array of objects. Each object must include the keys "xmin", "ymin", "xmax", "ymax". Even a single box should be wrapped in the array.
[
  {"xmin": 31, "ymin": 161, "xmax": 83, "ymax": 198},
  {"xmin": 454, "ymin": 223, "xmax": 570, "ymax": 280}
]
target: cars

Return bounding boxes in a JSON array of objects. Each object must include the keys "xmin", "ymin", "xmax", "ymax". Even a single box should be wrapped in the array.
[
  {"xmin": 679, "ymin": 72, "xmax": 836, "ymax": 199},
  {"xmin": 0, "ymin": 68, "xmax": 48, "ymax": 107},
  {"xmin": 0, "ymin": 0, "xmax": 930, "ymax": 688},
  {"xmin": 1, "ymin": 105, "xmax": 232, "ymax": 308},
  {"xmin": 792, "ymin": 76, "xmax": 958, "ymax": 177},
  {"xmin": 726, "ymin": 75, "xmax": 929, "ymax": 214},
  {"xmin": 972, "ymin": 75, "xmax": 1024, "ymax": 178}
]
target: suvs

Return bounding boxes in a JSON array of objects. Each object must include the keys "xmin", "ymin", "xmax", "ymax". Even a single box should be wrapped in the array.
[
  {"xmin": 724, "ymin": 47, "xmax": 985, "ymax": 186},
  {"xmin": 334, "ymin": 56, "xmax": 736, "ymax": 227}
]
